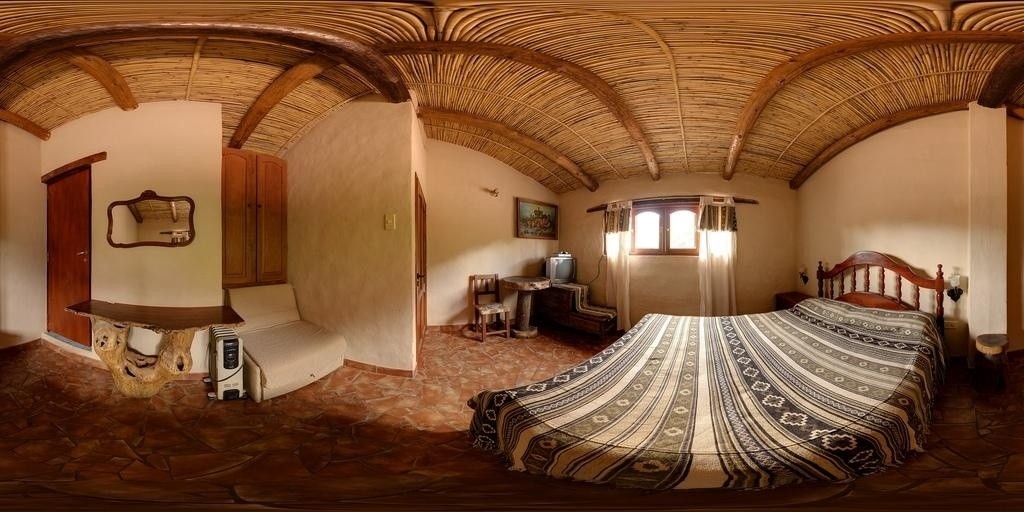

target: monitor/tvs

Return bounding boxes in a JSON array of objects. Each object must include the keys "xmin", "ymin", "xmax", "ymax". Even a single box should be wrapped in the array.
[{"xmin": 545, "ymin": 256, "xmax": 575, "ymax": 284}]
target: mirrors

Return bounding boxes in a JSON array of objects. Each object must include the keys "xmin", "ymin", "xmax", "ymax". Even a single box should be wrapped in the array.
[{"xmin": 106, "ymin": 190, "xmax": 195, "ymax": 248}]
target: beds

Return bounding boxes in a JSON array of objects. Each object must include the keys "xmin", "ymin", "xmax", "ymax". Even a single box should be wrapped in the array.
[{"xmin": 468, "ymin": 251, "xmax": 945, "ymax": 491}]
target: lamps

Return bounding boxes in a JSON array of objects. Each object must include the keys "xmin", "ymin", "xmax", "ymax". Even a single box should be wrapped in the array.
[
  {"xmin": 947, "ymin": 275, "xmax": 963, "ymax": 303},
  {"xmin": 797, "ymin": 265, "xmax": 809, "ymax": 285}
]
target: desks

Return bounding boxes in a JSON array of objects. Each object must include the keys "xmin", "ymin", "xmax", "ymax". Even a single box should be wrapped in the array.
[
  {"xmin": 63, "ymin": 300, "xmax": 246, "ymax": 399},
  {"xmin": 502, "ymin": 276, "xmax": 551, "ymax": 339}
]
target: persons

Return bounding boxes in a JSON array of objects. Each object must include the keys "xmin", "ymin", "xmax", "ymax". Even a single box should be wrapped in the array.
[{"xmin": 524, "ymin": 209, "xmax": 551, "ymax": 235}]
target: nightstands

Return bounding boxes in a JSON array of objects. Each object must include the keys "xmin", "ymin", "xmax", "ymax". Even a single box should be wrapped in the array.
[{"xmin": 776, "ymin": 291, "xmax": 813, "ymax": 310}]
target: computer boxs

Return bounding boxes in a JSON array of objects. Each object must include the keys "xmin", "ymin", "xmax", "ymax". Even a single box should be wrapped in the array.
[{"xmin": 208, "ymin": 325, "xmax": 243, "ymax": 401}]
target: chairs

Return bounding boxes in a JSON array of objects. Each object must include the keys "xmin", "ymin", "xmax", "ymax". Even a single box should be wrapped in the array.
[
  {"xmin": 225, "ymin": 285, "xmax": 347, "ymax": 404},
  {"xmin": 474, "ymin": 274, "xmax": 511, "ymax": 342}
]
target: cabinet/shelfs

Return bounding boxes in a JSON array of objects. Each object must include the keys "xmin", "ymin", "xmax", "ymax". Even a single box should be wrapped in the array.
[
  {"xmin": 537, "ymin": 283, "xmax": 617, "ymax": 346},
  {"xmin": 222, "ymin": 147, "xmax": 287, "ymax": 289}
]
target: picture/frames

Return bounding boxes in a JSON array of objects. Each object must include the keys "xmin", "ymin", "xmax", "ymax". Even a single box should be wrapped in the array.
[{"xmin": 516, "ymin": 197, "xmax": 558, "ymax": 240}]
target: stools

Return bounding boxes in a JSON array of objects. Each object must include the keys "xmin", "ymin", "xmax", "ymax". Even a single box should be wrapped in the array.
[{"xmin": 975, "ymin": 334, "xmax": 1009, "ymax": 393}]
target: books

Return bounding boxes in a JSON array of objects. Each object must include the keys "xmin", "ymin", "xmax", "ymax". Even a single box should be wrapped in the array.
[{"xmin": 551, "ymin": 253, "xmax": 572, "ymax": 256}]
